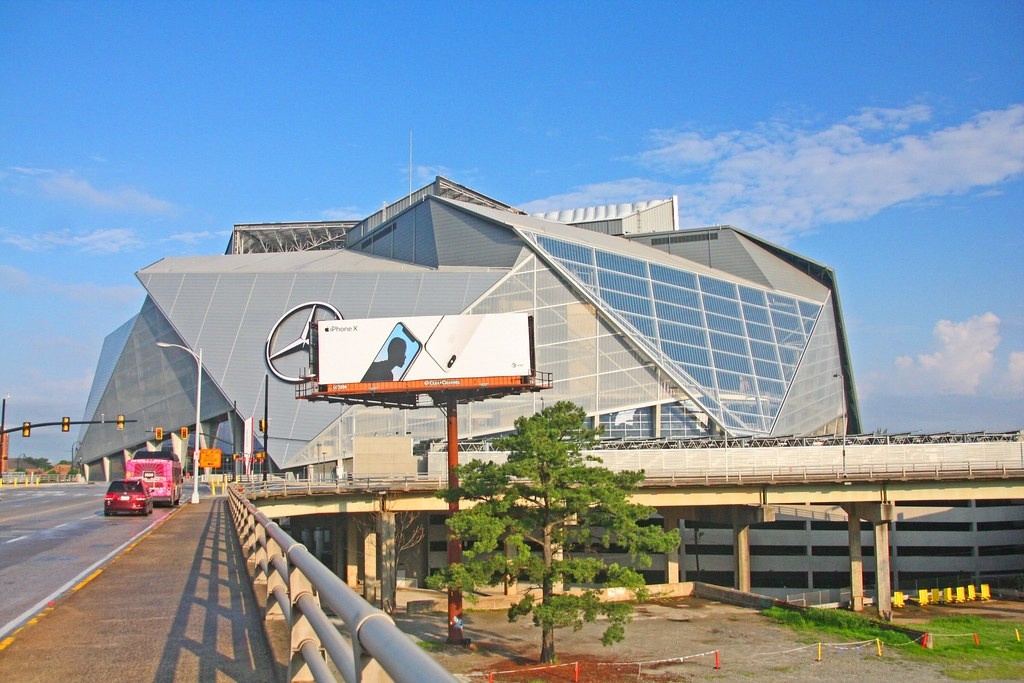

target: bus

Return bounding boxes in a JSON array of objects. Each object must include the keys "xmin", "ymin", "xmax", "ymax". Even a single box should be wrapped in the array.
[{"xmin": 125, "ymin": 450, "xmax": 183, "ymax": 508}]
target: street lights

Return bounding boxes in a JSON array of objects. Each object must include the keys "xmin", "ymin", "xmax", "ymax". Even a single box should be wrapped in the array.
[
  {"xmin": 694, "ymin": 528, "xmax": 704, "ymax": 581},
  {"xmin": 156, "ymin": 342, "xmax": 202, "ymax": 504},
  {"xmin": 317, "ymin": 444, "xmax": 322, "ymax": 465},
  {"xmin": 540, "ymin": 397, "xmax": 544, "ymax": 409},
  {"xmin": 833, "ymin": 374, "xmax": 846, "ymax": 475},
  {"xmin": 225, "ymin": 458, "xmax": 229, "ymax": 469},
  {"xmin": 17, "ymin": 453, "xmax": 25, "ymax": 471},
  {"xmin": 323, "ymin": 452, "xmax": 327, "ymax": 482},
  {"xmin": 242, "ymin": 453, "xmax": 248, "ymax": 474},
  {"xmin": 72, "ymin": 441, "xmax": 80, "ymax": 474}
]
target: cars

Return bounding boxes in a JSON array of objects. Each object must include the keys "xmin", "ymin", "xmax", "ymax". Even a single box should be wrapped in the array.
[{"xmin": 104, "ymin": 479, "xmax": 153, "ymax": 516}]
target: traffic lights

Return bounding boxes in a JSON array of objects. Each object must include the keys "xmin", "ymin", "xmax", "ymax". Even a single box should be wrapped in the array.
[
  {"xmin": 156, "ymin": 428, "xmax": 162, "ymax": 439},
  {"xmin": 259, "ymin": 420, "xmax": 264, "ymax": 432},
  {"xmin": 182, "ymin": 428, "xmax": 187, "ymax": 439}
]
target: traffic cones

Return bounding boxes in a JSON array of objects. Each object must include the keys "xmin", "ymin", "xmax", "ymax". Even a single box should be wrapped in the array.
[
  {"xmin": 217, "ymin": 477, "xmax": 220, "ymax": 485},
  {"xmin": 0, "ymin": 478, "xmax": 3, "ymax": 487},
  {"xmin": 222, "ymin": 483, "xmax": 225, "ymax": 492},
  {"xmin": 13, "ymin": 478, "xmax": 18, "ymax": 486},
  {"xmin": 211, "ymin": 477, "xmax": 214, "ymax": 484},
  {"xmin": 24, "ymin": 478, "xmax": 28, "ymax": 486},
  {"xmin": 36, "ymin": 478, "xmax": 40, "ymax": 486},
  {"xmin": 212, "ymin": 484, "xmax": 216, "ymax": 495}
]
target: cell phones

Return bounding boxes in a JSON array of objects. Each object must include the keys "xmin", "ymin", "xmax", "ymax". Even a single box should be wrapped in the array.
[
  {"xmin": 359, "ymin": 322, "xmax": 423, "ymax": 383},
  {"xmin": 425, "ymin": 315, "xmax": 485, "ymax": 372}
]
